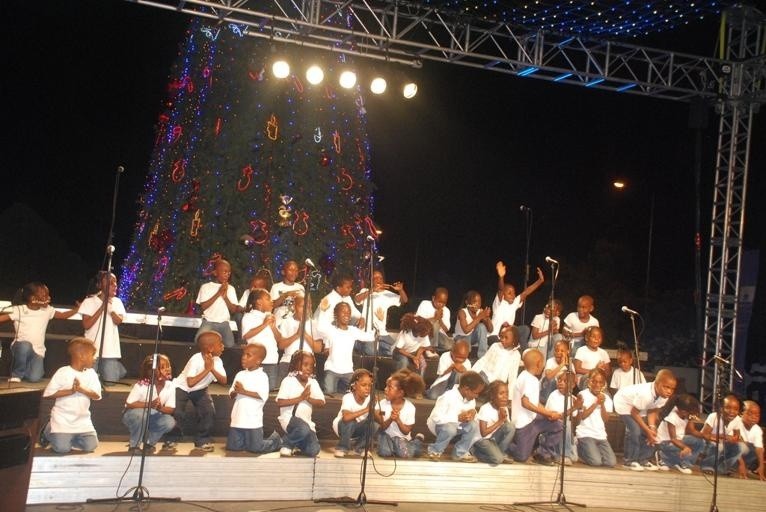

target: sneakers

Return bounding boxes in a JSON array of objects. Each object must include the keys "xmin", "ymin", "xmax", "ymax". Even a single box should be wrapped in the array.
[
  {"xmin": 424, "ymin": 348, "xmax": 439, "ymax": 360},
  {"xmin": 7, "ymin": 376, "xmax": 22, "ymax": 383}
]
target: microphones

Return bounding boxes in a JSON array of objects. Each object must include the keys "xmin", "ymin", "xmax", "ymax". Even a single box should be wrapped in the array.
[
  {"xmin": 545, "ymin": 256, "xmax": 558, "ymax": 264},
  {"xmin": 117, "ymin": 166, "xmax": 125, "ymax": 173},
  {"xmin": 157, "ymin": 306, "xmax": 165, "ymax": 320},
  {"xmin": 366, "ymin": 235, "xmax": 375, "ymax": 242},
  {"xmin": 520, "ymin": 205, "xmax": 530, "ymax": 211},
  {"xmin": 305, "ymin": 258, "xmax": 318, "ymax": 271},
  {"xmin": 622, "ymin": 306, "xmax": 638, "ymax": 315},
  {"xmin": 714, "ymin": 355, "xmax": 730, "ymax": 364},
  {"xmin": 563, "ymin": 327, "xmax": 573, "ymax": 334},
  {"xmin": 374, "ymin": 329, "xmax": 380, "ymax": 351},
  {"xmin": 106, "ymin": 245, "xmax": 115, "ymax": 271}
]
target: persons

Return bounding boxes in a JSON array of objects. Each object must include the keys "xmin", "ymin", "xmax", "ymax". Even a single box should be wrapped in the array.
[
  {"xmin": 699, "ymin": 394, "xmax": 748, "ymax": 480},
  {"xmin": 119, "ymin": 351, "xmax": 177, "ymax": 454},
  {"xmin": 237, "ymin": 260, "xmax": 648, "ymax": 392},
  {"xmin": 77, "ymin": 273, "xmax": 128, "ymax": 383},
  {"xmin": 0, "ymin": 283, "xmax": 78, "ymax": 383},
  {"xmin": 196, "ymin": 260, "xmax": 238, "ymax": 347},
  {"xmin": 657, "ymin": 392, "xmax": 711, "ymax": 478},
  {"xmin": 737, "ymin": 399, "xmax": 766, "ymax": 481},
  {"xmin": 332, "ymin": 369, "xmax": 382, "ymax": 457},
  {"xmin": 511, "ymin": 345, "xmax": 566, "ymax": 463},
  {"xmin": 578, "ymin": 368, "xmax": 619, "ymax": 469},
  {"xmin": 274, "ymin": 348, "xmax": 327, "ymax": 457},
  {"xmin": 613, "ymin": 366, "xmax": 679, "ymax": 471},
  {"xmin": 545, "ymin": 369, "xmax": 584, "ymax": 463},
  {"xmin": 470, "ymin": 379, "xmax": 518, "ymax": 467},
  {"xmin": 36, "ymin": 337, "xmax": 104, "ymax": 455},
  {"xmin": 161, "ymin": 329, "xmax": 227, "ymax": 452},
  {"xmin": 225, "ymin": 342, "xmax": 283, "ymax": 456},
  {"xmin": 373, "ymin": 368, "xmax": 427, "ymax": 460},
  {"xmin": 423, "ymin": 369, "xmax": 487, "ymax": 462}
]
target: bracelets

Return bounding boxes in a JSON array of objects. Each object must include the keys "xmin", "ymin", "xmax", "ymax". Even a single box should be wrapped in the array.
[{"xmin": 648, "ymin": 423, "xmax": 657, "ymax": 429}]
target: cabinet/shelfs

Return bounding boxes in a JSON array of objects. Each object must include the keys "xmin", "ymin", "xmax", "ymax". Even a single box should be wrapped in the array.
[{"xmin": 0, "ymin": 380, "xmax": 46, "ymax": 511}]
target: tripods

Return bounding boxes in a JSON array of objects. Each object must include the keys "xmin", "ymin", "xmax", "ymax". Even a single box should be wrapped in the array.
[
  {"xmin": 513, "ymin": 343, "xmax": 586, "ymax": 512},
  {"xmin": 94, "ymin": 273, "xmax": 133, "ymax": 394},
  {"xmin": 87, "ymin": 323, "xmax": 181, "ymax": 512},
  {"xmin": 314, "ymin": 352, "xmax": 398, "ymax": 512},
  {"xmin": 268, "ymin": 277, "xmax": 335, "ymax": 399}
]
